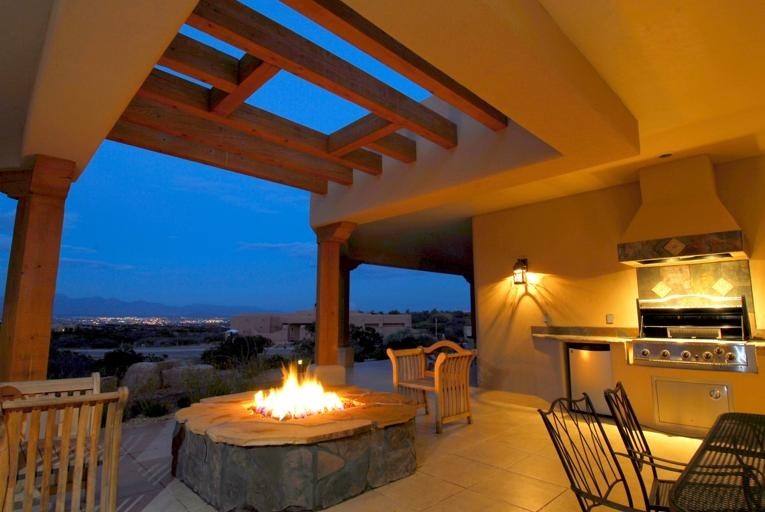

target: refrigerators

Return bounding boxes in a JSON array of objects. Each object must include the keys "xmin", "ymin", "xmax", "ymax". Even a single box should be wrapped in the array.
[{"xmin": 568, "ymin": 344, "xmax": 615, "ymax": 418}]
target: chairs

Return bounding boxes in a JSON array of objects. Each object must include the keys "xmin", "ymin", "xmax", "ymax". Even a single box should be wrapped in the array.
[
  {"xmin": 1, "ymin": 372, "xmax": 129, "ymax": 512},
  {"xmin": 538, "ymin": 381, "xmax": 765, "ymax": 512},
  {"xmin": 387, "ymin": 341, "xmax": 479, "ymax": 434}
]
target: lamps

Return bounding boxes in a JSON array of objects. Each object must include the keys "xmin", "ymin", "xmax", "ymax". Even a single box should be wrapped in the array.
[{"xmin": 512, "ymin": 257, "xmax": 528, "ymax": 284}]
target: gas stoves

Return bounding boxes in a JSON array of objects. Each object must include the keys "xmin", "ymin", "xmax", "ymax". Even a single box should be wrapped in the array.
[{"xmin": 630, "ymin": 337, "xmax": 759, "ymax": 373}]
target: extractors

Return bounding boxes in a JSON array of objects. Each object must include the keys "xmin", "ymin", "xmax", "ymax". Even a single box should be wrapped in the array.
[{"xmin": 617, "ymin": 155, "xmax": 752, "ymax": 269}]
